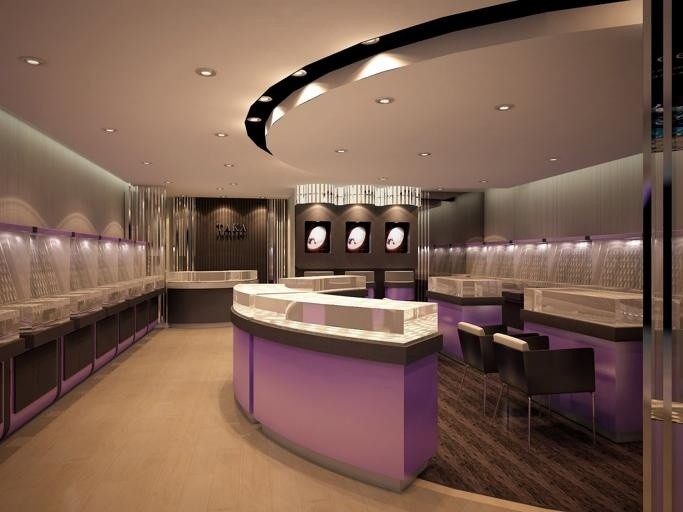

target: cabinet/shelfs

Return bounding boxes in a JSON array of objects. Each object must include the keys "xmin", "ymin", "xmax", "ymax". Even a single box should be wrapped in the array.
[
  {"xmin": 230, "ymin": 275, "xmax": 444, "ymax": 493},
  {"xmin": 425, "ymin": 274, "xmax": 503, "ymax": 366},
  {"xmin": 164, "ymin": 270, "xmax": 259, "ymax": 329},
  {"xmin": 519, "ymin": 287, "xmax": 655, "ymax": 443},
  {"xmin": 344, "ymin": 270, "xmax": 375, "ymax": 298},
  {"xmin": 304, "ymin": 271, "xmax": 334, "ymax": 277},
  {"xmin": 491, "ymin": 332, "xmax": 597, "ymax": 451},
  {"xmin": 383, "ymin": 270, "xmax": 415, "ymax": 301},
  {"xmin": 1, "ymin": 276, "xmax": 166, "ymax": 439}
]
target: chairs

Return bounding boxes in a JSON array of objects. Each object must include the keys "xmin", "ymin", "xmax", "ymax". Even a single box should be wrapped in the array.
[{"xmin": 456, "ymin": 321, "xmax": 541, "ymax": 418}]
